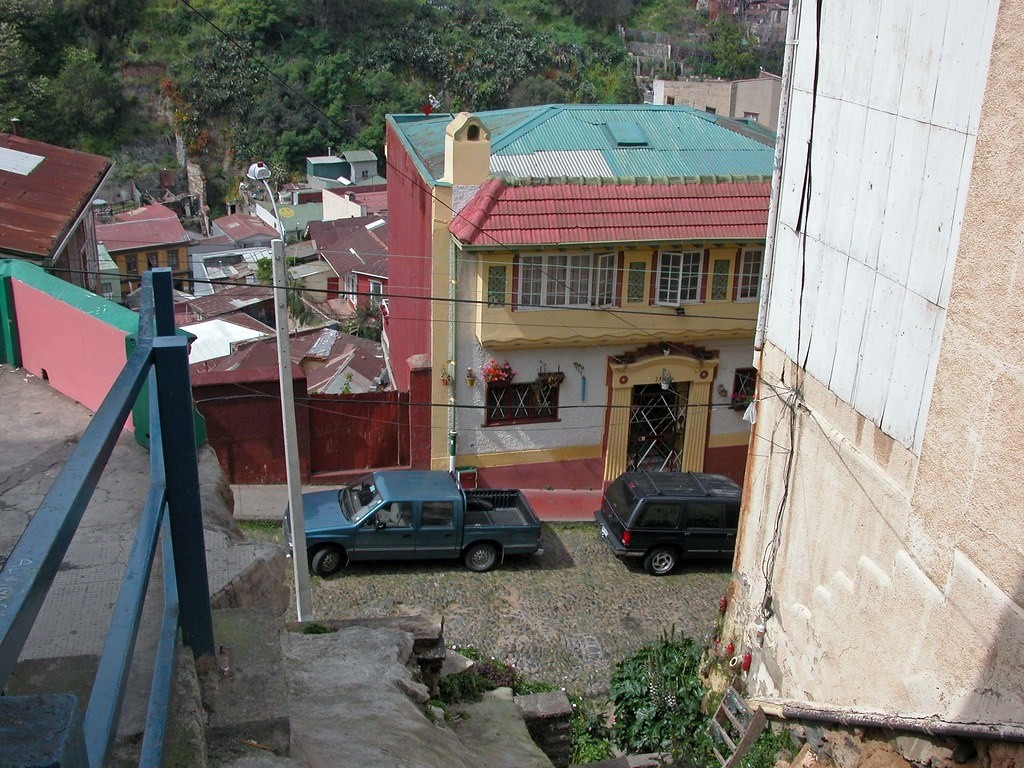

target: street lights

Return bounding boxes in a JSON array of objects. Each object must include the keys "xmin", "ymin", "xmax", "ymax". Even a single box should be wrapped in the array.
[{"xmin": 247, "ymin": 162, "xmax": 314, "ymax": 623}]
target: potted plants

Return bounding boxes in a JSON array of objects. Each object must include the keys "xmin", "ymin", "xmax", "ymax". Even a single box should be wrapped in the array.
[
  {"xmin": 733, "ymin": 403, "xmax": 751, "ymax": 411},
  {"xmin": 536, "ymin": 361, "xmax": 567, "ymax": 390},
  {"xmin": 438, "ymin": 364, "xmax": 452, "ymax": 387},
  {"xmin": 488, "ymin": 381, "xmax": 511, "ymax": 388},
  {"xmin": 464, "ymin": 366, "xmax": 476, "ymax": 387}
]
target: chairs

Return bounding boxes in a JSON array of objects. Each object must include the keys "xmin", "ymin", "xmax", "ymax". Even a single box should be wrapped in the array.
[{"xmin": 376, "ymin": 502, "xmax": 411, "ymax": 527}]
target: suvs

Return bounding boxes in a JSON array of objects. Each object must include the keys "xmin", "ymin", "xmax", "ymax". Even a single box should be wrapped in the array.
[{"xmin": 592, "ymin": 470, "xmax": 744, "ymax": 575}]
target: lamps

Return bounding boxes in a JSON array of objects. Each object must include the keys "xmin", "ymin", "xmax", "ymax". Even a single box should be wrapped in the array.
[{"xmin": 660, "ymin": 368, "xmax": 674, "ymax": 390}]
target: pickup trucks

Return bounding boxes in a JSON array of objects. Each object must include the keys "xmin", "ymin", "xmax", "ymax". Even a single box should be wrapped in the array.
[{"xmin": 283, "ymin": 470, "xmax": 543, "ymax": 572}]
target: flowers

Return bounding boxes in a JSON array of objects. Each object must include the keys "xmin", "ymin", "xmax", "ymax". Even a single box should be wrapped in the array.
[
  {"xmin": 480, "ymin": 360, "xmax": 518, "ymax": 382},
  {"xmin": 733, "ymin": 391, "xmax": 755, "ymax": 404}
]
